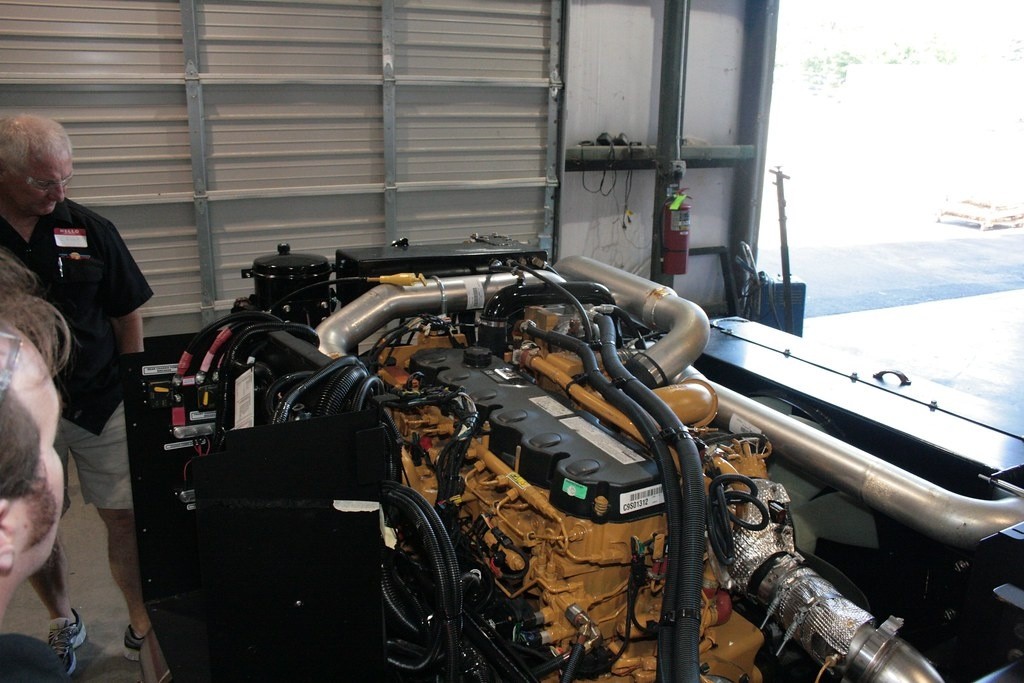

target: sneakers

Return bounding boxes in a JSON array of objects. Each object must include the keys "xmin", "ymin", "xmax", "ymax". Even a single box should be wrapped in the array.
[
  {"xmin": 122, "ymin": 623, "xmax": 146, "ymax": 661},
  {"xmin": 48, "ymin": 608, "xmax": 87, "ymax": 675}
]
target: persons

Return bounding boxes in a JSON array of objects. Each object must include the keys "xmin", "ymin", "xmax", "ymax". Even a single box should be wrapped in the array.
[
  {"xmin": 0, "ymin": 252, "xmax": 71, "ymax": 683},
  {"xmin": 0, "ymin": 114, "xmax": 153, "ymax": 676}
]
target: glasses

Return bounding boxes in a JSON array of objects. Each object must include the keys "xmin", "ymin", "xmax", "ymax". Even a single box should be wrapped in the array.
[{"xmin": 6, "ymin": 165, "xmax": 74, "ymax": 192}]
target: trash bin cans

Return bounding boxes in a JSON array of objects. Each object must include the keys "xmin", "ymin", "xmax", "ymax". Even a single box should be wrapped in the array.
[{"xmin": 660, "ymin": 187, "xmax": 693, "ymax": 274}]
